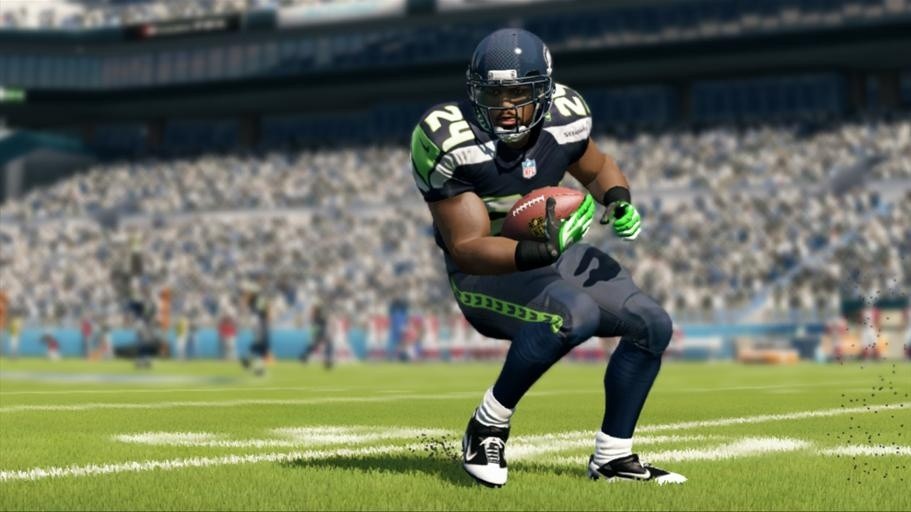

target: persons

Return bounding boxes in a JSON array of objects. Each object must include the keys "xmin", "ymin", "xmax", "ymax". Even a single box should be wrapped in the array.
[
  {"xmin": 0, "ymin": 1, "xmax": 911, "ymax": 363},
  {"xmin": 417, "ymin": 25, "xmax": 691, "ymax": 491}
]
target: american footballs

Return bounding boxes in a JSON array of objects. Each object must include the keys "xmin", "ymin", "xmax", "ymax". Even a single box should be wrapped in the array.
[{"xmin": 500, "ymin": 186, "xmax": 585, "ymax": 241}]
